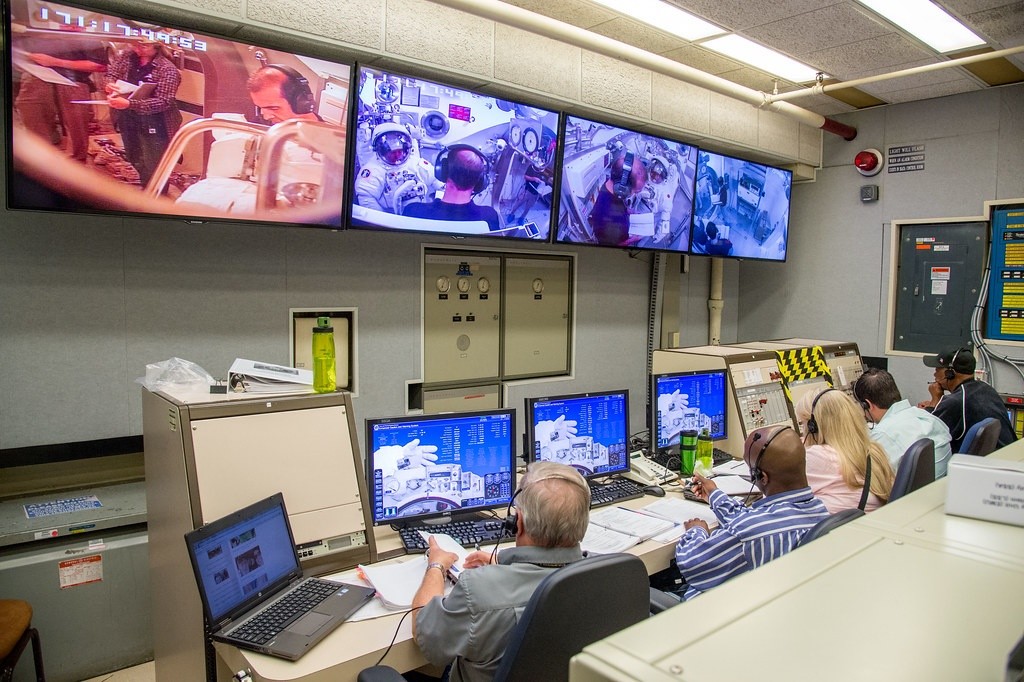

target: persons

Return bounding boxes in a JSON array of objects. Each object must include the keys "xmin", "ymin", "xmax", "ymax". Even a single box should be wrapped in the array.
[
  {"xmin": 215, "ymin": 573, "xmax": 225, "ymax": 584},
  {"xmin": 246, "ymin": 65, "xmax": 324, "ymax": 162},
  {"xmin": 250, "ymin": 548, "xmax": 263, "ymax": 570},
  {"xmin": 917, "ymin": 346, "xmax": 1019, "ymax": 456},
  {"xmin": 589, "ymin": 138, "xmax": 680, "ymax": 247},
  {"xmin": 712, "ymin": 176, "xmax": 730, "ymax": 224},
  {"xmin": 355, "ymin": 122, "xmax": 445, "ymax": 216},
  {"xmin": 855, "ymin": 367, "xmax": 952, "ymax": 482},
  {"xmin": 797, "ymin": 385, "xmax": 896, "ymax": 517},
  {"xmin": 507, "ymin": 166, "xmax": 548, "ymax": 226},
  {"xmin": 412, "ymin": 460, "xmax": 603, "ymax": 682},
  {"xmin": 401, "ymin": 144, "xmax": 501, "ymax": 232},
  {"xmin": 705, "ymin": 222, "xmax": 736, "ymax": 257},
  {"xmin": 693, "ymin": 213, "xmax": 708, "ymax": 246},
  {"xmin": 102, "ymin": 38, "xmax": 184, "ymax": 196},
  {"xmin": 14, "ymin": 21, "xmax": 112, "ymax": 161},
  {"xmin": 664, "ymin": 425, "xmax": 829, "ymax": 602}
]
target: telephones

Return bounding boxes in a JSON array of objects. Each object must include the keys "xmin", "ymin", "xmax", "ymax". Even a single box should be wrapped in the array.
[{"xmin": 619, "ymin": 450, "xmax": 679, "ymax": 487}]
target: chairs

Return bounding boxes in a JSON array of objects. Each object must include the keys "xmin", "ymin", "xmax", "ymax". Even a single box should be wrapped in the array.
[
  {"xmin": 650, "ymin": 509, "xmax": 867, "ymax": 616},
  {"xmin": 958, "ymin": 417, "xmax": 1001, "ymax": 457},
  {"xmin": 886, "ymin": 438, "xmax": 935, "ymax": 502},
  {"xmin": 0, "ymin": 598, "xmax": 46, "ymax": 682},
  {"xmin": 356, "ymin": 552, "xmax": 650, "ymax": 682}
]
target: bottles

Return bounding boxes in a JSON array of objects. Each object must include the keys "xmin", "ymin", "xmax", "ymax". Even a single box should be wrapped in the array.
[
  {"xmin": 680, "ymin": 430, "xmax": 697, "ymax": 477},
  {"xmin": 696, "ymin": 427, "xmax": 713, "ymax": 470},
  {"xmin": 313, "ymin": 318, "xmax": 338, "ymax": 393}
]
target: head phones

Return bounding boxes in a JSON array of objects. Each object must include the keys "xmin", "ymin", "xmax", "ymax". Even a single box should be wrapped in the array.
[
  {"xmin": 751, "ymin": 426, "xmax": 791, "ymax": 484},
  {"xmin": 434, "ymin": 144, "xmax": 490, "ymax": 193},
  {"xmin": 944, "ymin": 347, "xmax": 964, "ymax": 380},
  {"xmin": 613, "ymin": 152, "xmax": 634, "ymax": 199},
  {"xmin": 807, "ymin": 388, "xmax": 837, "ymax": 434},
  {"xmin": 504, "ymin": 475, "xmax": 592, "ymax": 536},
  {"xmin": 853, "ymin": 379, "xmax": 870, "ymax": 409},
  {"xmin": 267, "ymin": 63, "xmax": 317, "ymax": 115}
]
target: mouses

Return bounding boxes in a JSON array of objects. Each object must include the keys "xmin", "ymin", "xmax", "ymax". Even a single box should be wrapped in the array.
[{"xmin": 642, "ymin": 485, "xmax": 666, "ymax": 498}]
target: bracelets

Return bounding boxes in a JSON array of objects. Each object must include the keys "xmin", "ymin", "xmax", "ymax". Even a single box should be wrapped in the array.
[{"xmin": 426, "ymin": 563, "xmax": 447, "ymax": 580}]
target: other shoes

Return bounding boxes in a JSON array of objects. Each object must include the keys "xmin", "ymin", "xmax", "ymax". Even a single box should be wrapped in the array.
[{"xmin": 50, "ymin": 131, "xmax": 61, "ymax": 145}]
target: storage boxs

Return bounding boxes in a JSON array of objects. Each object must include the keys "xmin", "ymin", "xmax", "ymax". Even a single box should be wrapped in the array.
[{"xmin": 945, "ymin": 453, "xmax": 1024, "ymax": 528}]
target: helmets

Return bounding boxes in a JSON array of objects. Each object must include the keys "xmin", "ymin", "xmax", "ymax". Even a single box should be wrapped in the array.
[
  {"xmin": 371, "ymin": 122, "xmax": 413, "ymax": 169},
  {"xmin": 648, "ymin": 155, "xmax": 671, "ymax": 186}
]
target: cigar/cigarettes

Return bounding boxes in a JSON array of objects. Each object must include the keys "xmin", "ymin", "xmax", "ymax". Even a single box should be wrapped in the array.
[{"xmin": 927, "ymin": 382, "xmax": 936, "ymax": 384}]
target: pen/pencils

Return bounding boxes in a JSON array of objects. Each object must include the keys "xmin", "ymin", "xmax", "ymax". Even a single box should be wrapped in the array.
[
  {"xmin": 927, "ymin": 382, "xmax": 937, "ymax": 384},
  {"xmin": 475, "ymin": 543, "xmax": 487, "ymax": 566},
  {"xmin": 689, "ymin": 473, "xmax": 716, "ymax": 486}
]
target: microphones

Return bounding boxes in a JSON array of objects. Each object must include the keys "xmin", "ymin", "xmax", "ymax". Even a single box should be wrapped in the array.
[
  {"xmin": 749, "ymin": 434, "xmax": 761, "ymax": 472},
  {"xmin": 866, "ymin": 408, "xmax": 874, "ymax": 430},
  {"xmin": 928, "ymin": 378, "xmax": 945, "ymax": 384}
]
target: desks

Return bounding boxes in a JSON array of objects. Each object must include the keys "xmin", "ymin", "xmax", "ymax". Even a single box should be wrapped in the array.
[
  {"xmin": 567, "ymin": 437, "xmax": 1024, "ymax": 682},
  {"xmin": 211, "ymin": 460, "xmax": 768, "ymax": 682}
]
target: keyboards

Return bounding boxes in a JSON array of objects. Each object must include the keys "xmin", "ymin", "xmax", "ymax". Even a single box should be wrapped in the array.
[
  {"xmin": 649, "ymin": 448, "xmax": 732, "ymax": 470},
  {"xmin": 399, "ymin": 520, "xmax": 516, "ymax": 553},
  {"xmin": 589, "ymin": 479, "xmax": 645, "ymax": 509}
]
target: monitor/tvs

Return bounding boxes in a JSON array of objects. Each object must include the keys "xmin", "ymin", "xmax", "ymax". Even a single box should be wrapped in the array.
[
  {"xmin": 652, "ymin": 369, "xmax": 728, "ymax": 456},
  {"xmin": 524, "ymin": 389, "xmax": 631, "ymax": 486},
  {"xmin": 364, "ymin": 408, "xmax": 517, "ymax": 530},
  {"xmin": 1, "ymin": 1, "xmax": 793, "ymax": 263}
]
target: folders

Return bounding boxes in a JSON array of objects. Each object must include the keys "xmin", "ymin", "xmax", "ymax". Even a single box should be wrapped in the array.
[{"xmin": 226, "ymin": 358, "xmax": 314, "ymax": 396}]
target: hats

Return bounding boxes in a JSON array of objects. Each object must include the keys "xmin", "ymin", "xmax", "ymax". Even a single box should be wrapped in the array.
[{"xmin": 923, "ymin": 347, "xmax": 976, "ymax": 374}]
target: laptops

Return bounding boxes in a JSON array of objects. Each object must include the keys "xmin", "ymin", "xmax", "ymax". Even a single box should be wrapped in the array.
[{"xmin": 184, "ymin": 492, "xmax": 376, "ymax": 660}]
